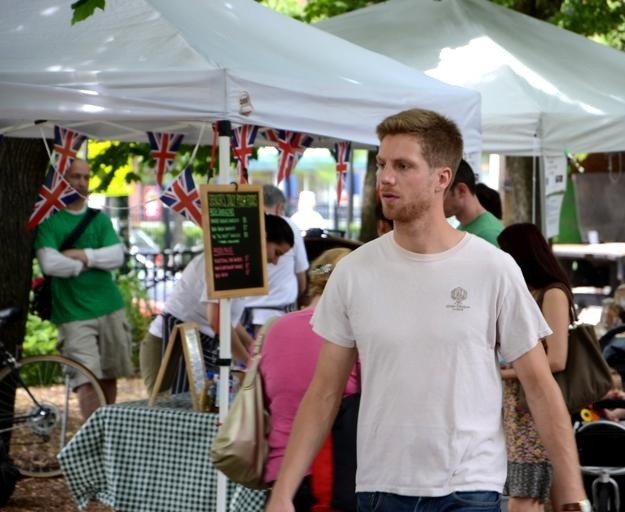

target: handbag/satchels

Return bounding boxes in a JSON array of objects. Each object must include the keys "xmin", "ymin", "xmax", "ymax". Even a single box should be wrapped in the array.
[
  {"xmin": 28, "ymin": 276, "xmax": 52, "ymax": 320},
  {"xmin": 209, "ymin": 354, "xmax": 270, "ymax": 490},
  {"xmin": 540, "ymin": 324, "xmax": 614, "ymax": 413}
]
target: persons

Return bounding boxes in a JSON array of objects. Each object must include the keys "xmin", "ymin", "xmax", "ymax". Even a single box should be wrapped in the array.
[
  {"xmin": 442, "ymin": 156, "xmax": 507, "ymax": 250},
  {"xmin": 265, "ymin": 110, "xmax": 591, "ymax": 511},
  {"xmin": 33, "ymin": 157, "xmax": 135, "ymax": 420},
  {"xmin": 144, "ymin": 185, "xmax": 623, "ymax": 512}
]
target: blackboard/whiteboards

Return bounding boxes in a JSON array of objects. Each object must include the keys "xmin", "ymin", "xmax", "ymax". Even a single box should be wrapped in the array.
[{"xmin": 200, "ymin": 184, "xmax": 268, "ymax": 298}]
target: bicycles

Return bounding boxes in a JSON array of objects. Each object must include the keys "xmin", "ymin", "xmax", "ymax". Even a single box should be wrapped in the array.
[{"xmin": 0, "ymin": 307, "xmax": 109, "ymax": 479}]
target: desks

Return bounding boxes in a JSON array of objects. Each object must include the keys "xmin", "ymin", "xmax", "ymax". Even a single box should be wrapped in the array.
[{"xmin": 100, "ymin": 392, "xmax": 267, "ymax": 512}]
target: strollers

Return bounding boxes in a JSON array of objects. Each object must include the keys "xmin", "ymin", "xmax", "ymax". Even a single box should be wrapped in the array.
[{"xmin": 570, "ymin": 325, "xmax": 625, "ymax": 512}]
